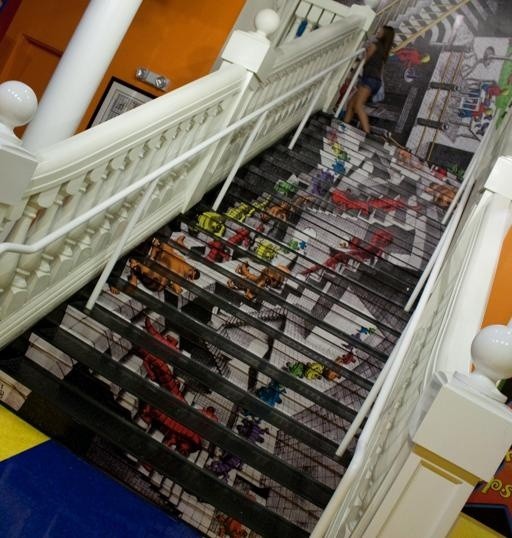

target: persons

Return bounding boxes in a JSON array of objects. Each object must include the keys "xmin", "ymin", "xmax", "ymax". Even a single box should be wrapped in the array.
[{"xmin": 342, "ymin": 26, "xmax": 394, "ymax": 136}]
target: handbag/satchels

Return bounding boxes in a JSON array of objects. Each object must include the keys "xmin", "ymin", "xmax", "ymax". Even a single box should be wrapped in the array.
[{"xmin": 372, "ymin": 81, "xmax": 385, "ymax": 103}]
